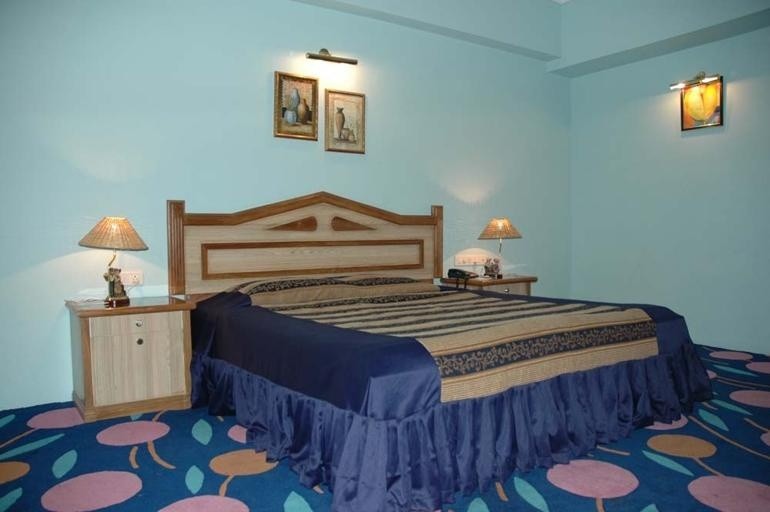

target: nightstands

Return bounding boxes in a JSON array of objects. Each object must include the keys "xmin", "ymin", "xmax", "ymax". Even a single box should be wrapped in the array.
[
  {"xmin": 439, "ymin": 272, "xmax": 539, "ymax": 296},
  {"xmin": 64, "ymin": 294, "xmax": 193, "ymax": 422}
]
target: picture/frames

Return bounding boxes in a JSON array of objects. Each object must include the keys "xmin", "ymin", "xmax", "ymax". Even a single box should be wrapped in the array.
[
  {"xmin": 322, "ymin": 87, "xmax": 367, "ymax": 155},
  {"xmin": 678, "ymin": 74, "xmax": 726, "ymax": 132},
  {"xmin": 272, "ymin": 70, "xmax": 320, "ymax": 143}
]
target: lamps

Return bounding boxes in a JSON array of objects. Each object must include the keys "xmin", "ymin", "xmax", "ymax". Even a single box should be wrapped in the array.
[
  {"xmin": 477, "ymin": 215, "xmax": 524, "ymax": 257},
  {"xmin": 77, "ymin": 215, "xmax": 149, "ymax": 267},
  {"xmin": 303, "ymin": 48, "xmax": 358, "ymax": 67},
  {"xmin": 669, "ymin": 69, "xmax": 724, "ymax": 91}
]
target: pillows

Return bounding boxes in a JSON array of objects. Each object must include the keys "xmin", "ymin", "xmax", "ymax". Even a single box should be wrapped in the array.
[{"xmin": 189, "ymin": 274, "xmax": 461, "ymax": 307}]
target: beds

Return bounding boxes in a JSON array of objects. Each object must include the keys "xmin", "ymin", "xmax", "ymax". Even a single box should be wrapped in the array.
[{"xmin": 165, "ymin": 189, "xmax": 718, "ymax": 510}]
